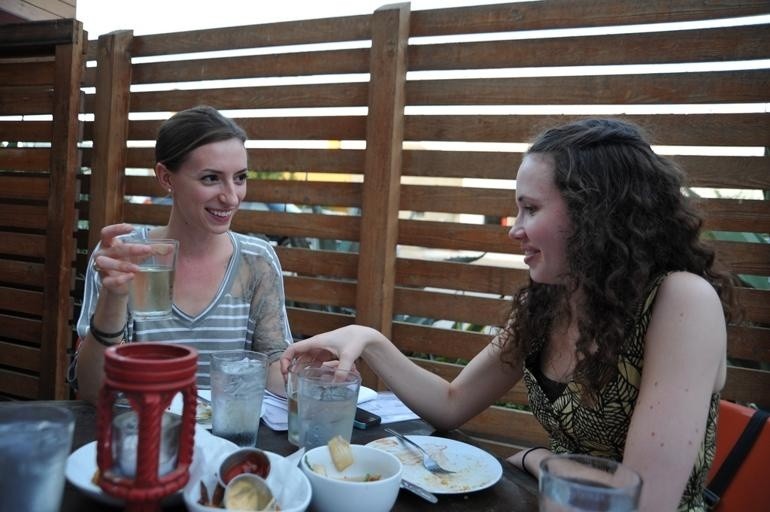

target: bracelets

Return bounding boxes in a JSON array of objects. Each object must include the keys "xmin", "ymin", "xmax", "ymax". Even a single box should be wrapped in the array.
[
  {"xmin": 89, "ymin": 307, "xmax": 136, "ymax": 346},
  {"xmin": 522, "ymin": 446, "xmax": 549, "ymax": 472}
]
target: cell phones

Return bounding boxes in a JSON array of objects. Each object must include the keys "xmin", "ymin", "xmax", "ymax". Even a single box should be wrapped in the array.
[{"xmin": 353, "ymin": 406, "xmax": 381, "ymax": 430}]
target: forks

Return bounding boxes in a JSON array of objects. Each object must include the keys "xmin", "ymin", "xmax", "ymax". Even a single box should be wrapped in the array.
[{"xmin": 382, "ymin": 425, "xmax": 456, "ymax": 476}]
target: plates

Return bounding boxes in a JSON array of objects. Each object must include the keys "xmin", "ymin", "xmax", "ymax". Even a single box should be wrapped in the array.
[
  {"xmin": 365, "ymin": 434, "xmax": 505, "ymax": 496},
  {"xmin": 169, "ymin": 390, "xmax": 267, "ymax": 431},
  {"xmin": 62, "ymin": 434, "xmax": 243, "ymax": 507}
]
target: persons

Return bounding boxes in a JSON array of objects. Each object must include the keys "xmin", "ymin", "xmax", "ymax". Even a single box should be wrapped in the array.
[
  {"xmin": 281, "ymin": 116, "xmax": 745, "ymax": 512},
  {"xmin": 75, "ymin": 104, "xmax": 293, "ymax": 404}
]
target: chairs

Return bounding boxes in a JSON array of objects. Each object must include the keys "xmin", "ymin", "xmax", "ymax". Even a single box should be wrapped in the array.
[{"xmin": 700, "ymin": 393, "xmax": 770, "ymax": 511}]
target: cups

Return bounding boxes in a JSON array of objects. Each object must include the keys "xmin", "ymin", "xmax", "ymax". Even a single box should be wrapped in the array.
[
  {"xmin": 535, "ymin": 453, "xmax": 644, "ymax": 511},
  {"xmin": 1, "ymin": 401, "xmax": 77, "ymax": 512},
  {"xmin": 299, "ymin": 444, "xmax": 401, "ymax": 511},
  {"xmin": 286, "ymin": 363, "xmax": 337, "ymax": 446},
  {"xmin": 298, "ymin": 365, "xmax": 362, "ymax": 452},
  {"xmin": 111, "ymin": 411, "xmax": 183, "ymax": 477},
  {"xmin": 182, "ymin": 451, "xmax": 312, "ymax": 510},
  {"xmin": 211, "ymin": 349, "xmax": 270, "ymax": 447},
  {"xmin": 121, "ymin": 238, "xmax": 180, "ymax": 320}
]
